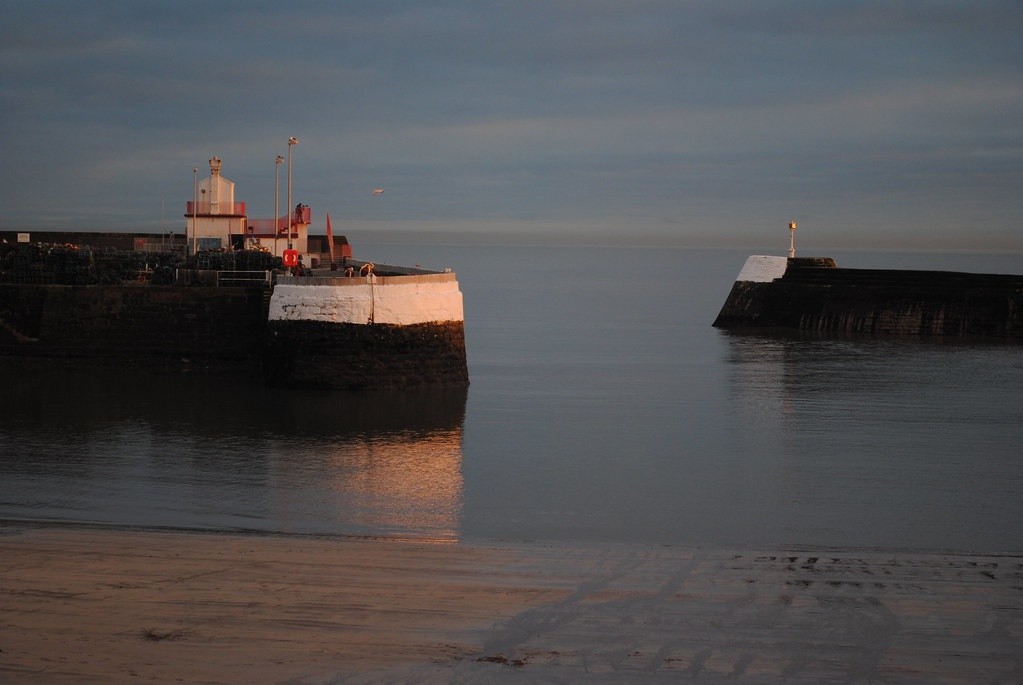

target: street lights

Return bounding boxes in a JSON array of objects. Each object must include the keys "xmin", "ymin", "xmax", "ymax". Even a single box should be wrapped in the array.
[
  {"xmin": 192, "ymin": 165, "xmax": 200, "ymax": 254},
  {"xmin": 285, "ymin": 136, "xmax": 298, "ymax": 276},
  {"xmin": 275, "ymin": 155, "xmax": 285, "ymax": 268}
]
